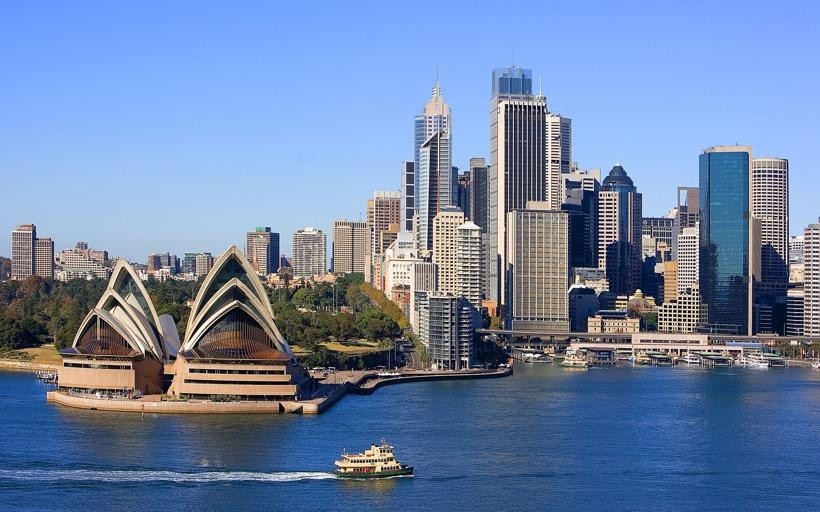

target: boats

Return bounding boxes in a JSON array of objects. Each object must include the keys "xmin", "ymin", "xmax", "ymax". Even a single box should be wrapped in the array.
[{"xmin": 557, "ymin": 353, "xmax": 593, "ymax": 369}]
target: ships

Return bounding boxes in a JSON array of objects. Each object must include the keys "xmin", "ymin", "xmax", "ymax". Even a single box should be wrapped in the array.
[{"xmin": 333, "ymin": 438, "xmax": 414, "ymax": 478}]
